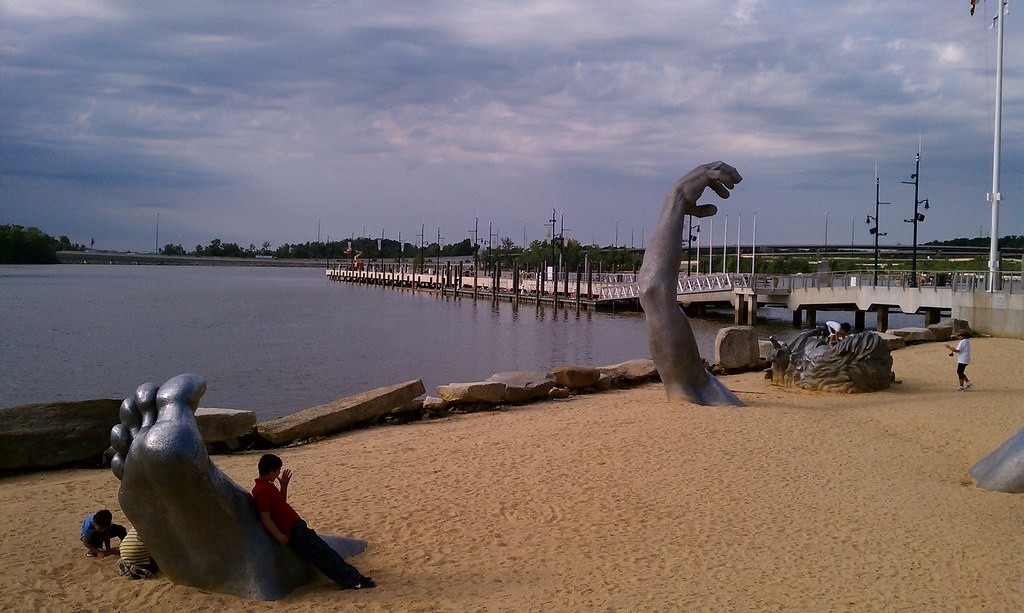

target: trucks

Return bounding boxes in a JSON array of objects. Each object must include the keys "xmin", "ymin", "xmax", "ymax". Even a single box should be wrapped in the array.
[{"xmin": 255, "ymin": 255, "xmax": 278, "ymax": 260}]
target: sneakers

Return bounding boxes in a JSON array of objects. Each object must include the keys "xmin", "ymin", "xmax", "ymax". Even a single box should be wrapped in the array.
[{"xmin": 347, "ymin": 577, "xmax": 375, "ymax": 589}]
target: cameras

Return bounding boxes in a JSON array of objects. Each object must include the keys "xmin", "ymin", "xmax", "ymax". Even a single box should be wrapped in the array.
[{"xmin": 949, "ymin": 353, "xmax": 953, "ymax": 356}]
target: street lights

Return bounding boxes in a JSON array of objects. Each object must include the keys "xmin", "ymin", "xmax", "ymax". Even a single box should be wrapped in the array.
[
  {"xmin": 421, "ymin": 240, "xmax": 428, "ymax": 273},
  {"xmin": 909, "ymin": 198, "xmax": 930, "ymax": 287},
  {"xmin": 552, "ymin": 233, "xmax": 564, "ymax": 281},
  {"xmin": 474, "ymin": 238, "xmax": 483, "ymax": 290},
  {"xmin": 484, "ymin": 240, "xmax": 491, "ymax": 276},
  {"xmin": 866, "ymin": 215, "xmax": 878, "ymax": 286},
  {"xmin": 687, "ymin": 225, "xmax": 700, "ymax": 275},
  {"xmin": 156, "ymin": 212, "xmax": 159, "ymax": 256}
]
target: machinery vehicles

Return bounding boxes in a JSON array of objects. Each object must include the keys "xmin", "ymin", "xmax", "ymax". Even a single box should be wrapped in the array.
[{"xmin": 342, "ymin": 246, "xmax": 365, "ymax": 271}]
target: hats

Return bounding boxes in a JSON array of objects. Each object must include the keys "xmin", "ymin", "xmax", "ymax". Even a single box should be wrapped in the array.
[{"xmin": 957, "ymin": 329, "xmax": 967, "ymax": 336}]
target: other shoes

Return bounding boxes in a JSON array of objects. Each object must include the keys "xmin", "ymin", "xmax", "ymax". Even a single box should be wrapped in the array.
[
  {"xmin": 86, "ymin": 550, "xmax": 96, "ymax": 557},
  {"xmin": 965, "ymin": 383, "xmax": 974, "ymax": 390},
  {"xmin": 954, "ymin": 387, "xmax": 965, "ymax": 392},
  {"xmin": 828, "ymin": 344, "xmax": 834, "ymax": 349},
  {"xmin": 130, "ymin": 565, "xmax": 154, "ymax": 578},
  {"xmin": 117, "ymin": 559, "xmax": 131, "ymax": 577}
]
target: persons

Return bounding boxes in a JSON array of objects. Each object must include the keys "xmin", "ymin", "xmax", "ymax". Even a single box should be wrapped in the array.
[
  {"xmin": 81, "ymin": 510, "xmax": 128, "ymax": 557},
  {"xmin": 521, "ymin": 286, "xmax": 525, "ymax": 295},
  {"xmin": 946, "ymin": 329, "xmax": 973, "ymax": 392},
  {"xmin": 824, "ymin": 321, "xmax": 850, "ymax": 347},
  {"xmin": 252, "ymin": 454, "xmax": 374, "ymax": 589},
  {"xmin": 118, "ymin": 527, "xmax": 158, "ymax": 579},
  {"xmin": 684, "ymin": 270, "xmax": 688, "ymax": 278},
  {"xmin": 481, "ymin": 283, "xmax": 484, "ymax": 290},
  {"xmin": 354, "ymin": 259, "xmax": 374, "ymax": 272},
  {"xmin": 105, "ymin": 161, "xmax": 1024, "ymax": 601},
  {"xmin": 400, "ymin": 264, "xmax": 405, "ymax": 274}
]
